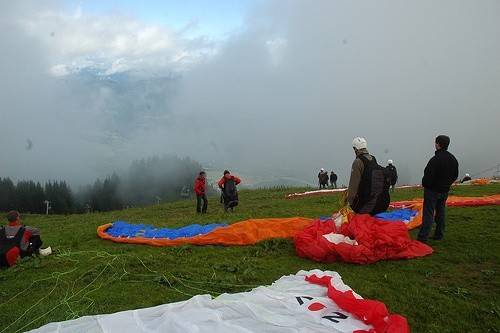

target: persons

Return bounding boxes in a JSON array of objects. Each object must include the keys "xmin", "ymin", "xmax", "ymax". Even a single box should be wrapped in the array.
[
  {"xmin": 194, "ymin": 171, "xmax": 209, "ymax": 215},
  {"xmin": 461, "ymin": 173, "xmax": 471, "ymax": 184},
  {"xmin": 218, "ymin": 170, "xmax": 241, "ymax": 213},
  {"xmin": 416, "ymin": 135, "xmax": 459, "ymax": 244},
  {"xmin": 318, "ymin": 168, "xmax": 329, "ymax": 189},
  {"xmin": 2, "ymin": 210, "xmax": 43, "ymax": 258},
  {"xmin": 345, "ymin": 137, "xmax": 378, "ymax": 216},
  {"xmin": 385, "ymin": 159, "xmax": 398, "ymax": 193},
  {"xmin": 330, "ymin": 171, "xmax": 338, "ymax": 188}
]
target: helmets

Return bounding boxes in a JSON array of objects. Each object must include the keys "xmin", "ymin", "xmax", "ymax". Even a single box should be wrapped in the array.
[
  {"xmin": 388, "ymin": 159, "xmax": 393, "ymax": 164},
  {"xmin": 352, "ymin": 137, "xmax": 368, "ymax": 150},
  {"xmin": 321, "ymin": 168, "xmax": 324, "ymax": 170}
]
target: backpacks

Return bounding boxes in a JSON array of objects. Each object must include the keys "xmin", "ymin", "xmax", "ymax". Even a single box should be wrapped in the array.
[
  {"xmin": 220, "ymin": 176, "xmax": 238, "ymax": 208},
  {"xmin": 350, "ymin": 155, "xmax": 391, "ymax": 216},
  {"xmin": 0, "ymin": 227, "xmax": 26, "ymax": 269}
]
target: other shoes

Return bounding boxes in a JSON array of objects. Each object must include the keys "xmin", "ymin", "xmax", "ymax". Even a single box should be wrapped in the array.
[
  {"xmin": 202, "ymin": 212, "xmax": 209, "ymax": 214},
  {"xmin": 428, "ymin": 235, "xmax": 441, "ymax": 241},
  {"xmin": 415, "ymin": 240, "xmax": 427, "ymax": 244},
  {"xmin": 198, "ymin": 212, "xmax": 201, "ymax": 215}
]
